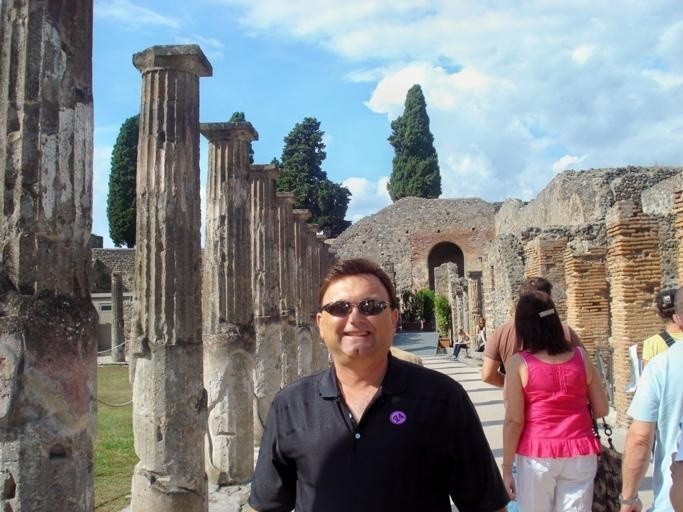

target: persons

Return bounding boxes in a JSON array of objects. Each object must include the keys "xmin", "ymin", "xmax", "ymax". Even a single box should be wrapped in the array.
[
  {"xmin": 451, "ymin": 327, "xmax": 471, "ymax": 358},
  {"xmin": 246, "ymin": 258, "xmax": 510, "ymax": 512},
  {"xmin": 479, "ymin": 277, "xmax": 683, "ymax": 512},
  {"xmin": 476, "ymin": 318, "xmax": 487, "ymax": 350}
]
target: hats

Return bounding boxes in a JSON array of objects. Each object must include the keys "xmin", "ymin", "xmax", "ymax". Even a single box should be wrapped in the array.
[{"xmin": 656, "ymin": 289, "xmax": 678, "ymax": 318}]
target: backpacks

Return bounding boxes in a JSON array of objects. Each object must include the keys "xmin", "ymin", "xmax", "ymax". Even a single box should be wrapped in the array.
[{"xmin": 623, "ymin": 331, "xmax": 675, "ymax": 393}]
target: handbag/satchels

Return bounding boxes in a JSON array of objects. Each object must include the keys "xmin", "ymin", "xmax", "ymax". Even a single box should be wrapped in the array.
[{"xmin": 591, "ymin": 444, "xmax": 622, "ymax": 512}]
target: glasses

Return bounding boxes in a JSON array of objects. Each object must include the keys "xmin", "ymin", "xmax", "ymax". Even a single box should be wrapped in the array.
[{"xmin": 320, "ymin": 299, "xmax": 392, "ymax": 316}]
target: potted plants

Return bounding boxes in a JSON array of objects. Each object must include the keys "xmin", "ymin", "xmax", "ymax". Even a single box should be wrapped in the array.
[
  {"xmin": 415, "ymin": 288, "xmax": 436, "ymax": 332},
  {"xmin": 436, "ymin": 293, "xmax": 452, "ymax": 349}
]
